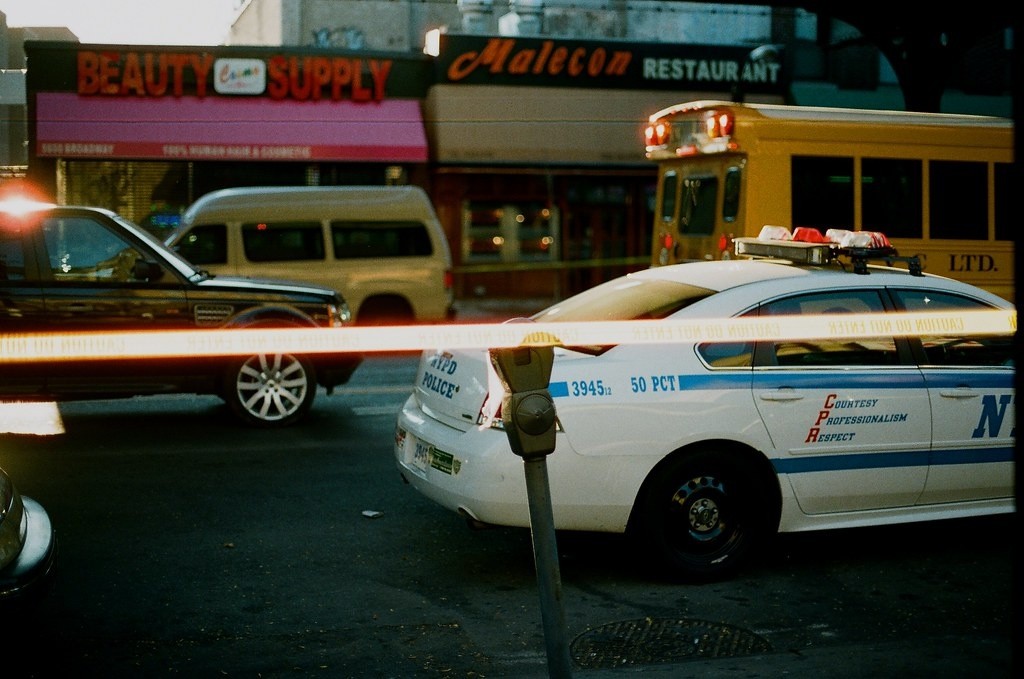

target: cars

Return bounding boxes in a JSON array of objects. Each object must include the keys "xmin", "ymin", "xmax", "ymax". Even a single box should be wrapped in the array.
[{"xmin": 392, "ymin": 226, "xmax": 1019, "ymax": 573}]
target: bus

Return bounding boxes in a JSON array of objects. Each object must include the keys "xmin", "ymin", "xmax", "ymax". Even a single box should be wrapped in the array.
[{"xmin": 645, "ymin": 99, "xmax": 1014, "ymax": 301}]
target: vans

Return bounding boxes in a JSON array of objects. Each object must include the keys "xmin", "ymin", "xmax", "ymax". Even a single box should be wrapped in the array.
[{"xmin": 165, "ymin": 186, "xmax": 453, "ymax": 324}]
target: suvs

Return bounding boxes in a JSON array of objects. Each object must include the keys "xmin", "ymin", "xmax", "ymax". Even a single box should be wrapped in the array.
[{"xmin": 2, "ymin": 203, "xmax": 363, "ymax": 425}]
